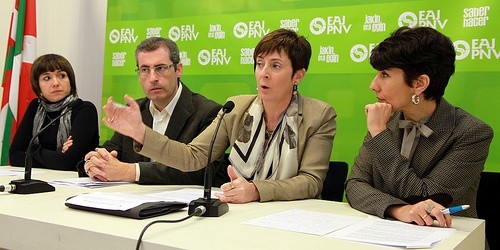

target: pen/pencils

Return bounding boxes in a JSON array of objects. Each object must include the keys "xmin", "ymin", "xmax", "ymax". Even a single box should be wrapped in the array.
[{"xmin": 429, "ymin": 205, "xmax": 470, "ymax": 217}]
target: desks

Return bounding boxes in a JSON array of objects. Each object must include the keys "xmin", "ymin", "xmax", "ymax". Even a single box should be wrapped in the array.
[{"xmin": 0, "ymin": 165, "xmax": 486, "ymax": 250}]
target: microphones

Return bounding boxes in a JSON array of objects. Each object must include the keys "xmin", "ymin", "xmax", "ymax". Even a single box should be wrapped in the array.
[
  {"xmin": 9, "ymin": 99, "xmax": 86, "ymax": 193},
  {"xmin": 188, "ymin": 100, "xmax": 236, "ymax": 215}
]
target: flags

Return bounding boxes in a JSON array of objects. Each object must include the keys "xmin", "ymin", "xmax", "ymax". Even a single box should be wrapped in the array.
[{"xmin": 0, "ymin": 0, "xmax": 38, "ymax": 167}]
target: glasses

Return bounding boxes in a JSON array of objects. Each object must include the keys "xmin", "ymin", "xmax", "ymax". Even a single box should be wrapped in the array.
[{"xmin": 135, "ymin": 62, "xmax": 176, "ymax": 77}]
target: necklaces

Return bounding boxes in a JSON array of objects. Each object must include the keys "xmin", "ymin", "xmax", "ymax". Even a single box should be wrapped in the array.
[{"xmin": 266, "ymin": 121, "xmax": 272, "ymax": 138}]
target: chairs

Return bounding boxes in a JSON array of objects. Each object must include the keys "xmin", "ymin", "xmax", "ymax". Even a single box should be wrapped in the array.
[{"xmin": 476, "ymin": 172, "xmax": 500, "ymax": 250}]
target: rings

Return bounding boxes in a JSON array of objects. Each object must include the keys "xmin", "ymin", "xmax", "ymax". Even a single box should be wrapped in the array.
[
  {"xmin": 63, "ymin": 149, "xmax": 64, "ymax": 151},
  {"xmin": 231, "ymin": 182, "xmax": 236, "ymax": 188},
  {"xmin": 423, "ymin": 214, "xmax": 427, "ymax": 219},
  {"xmin": 111, "ymin": 119, "xmax": 113, "ymax": 122},
  {"xmin": 232, "ymin": 196, "xmax": 234, "ymax": 202},
  {"xmin": 425, "ymin": 204, "xmax": 433, "ymax": 213},
  {"xmin": 86, "ymin": 161, "xmax": 89, "ymax": 164}
]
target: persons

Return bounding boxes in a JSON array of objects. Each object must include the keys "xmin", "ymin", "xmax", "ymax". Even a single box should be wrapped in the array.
[
  {"xmin": 8, "ymin": 54, "xmax": 100, "ymax": 170},
  {"xmin": 344, "ymin": 26, "xmax": 494, "ymax": 227},
  {"xmin": 102, "ymin": 28, "xmax": 337, "ymax": 203},
  {"xmin": 77, "ymin": 37, "xmax": 231, "ymax": 188}
]
